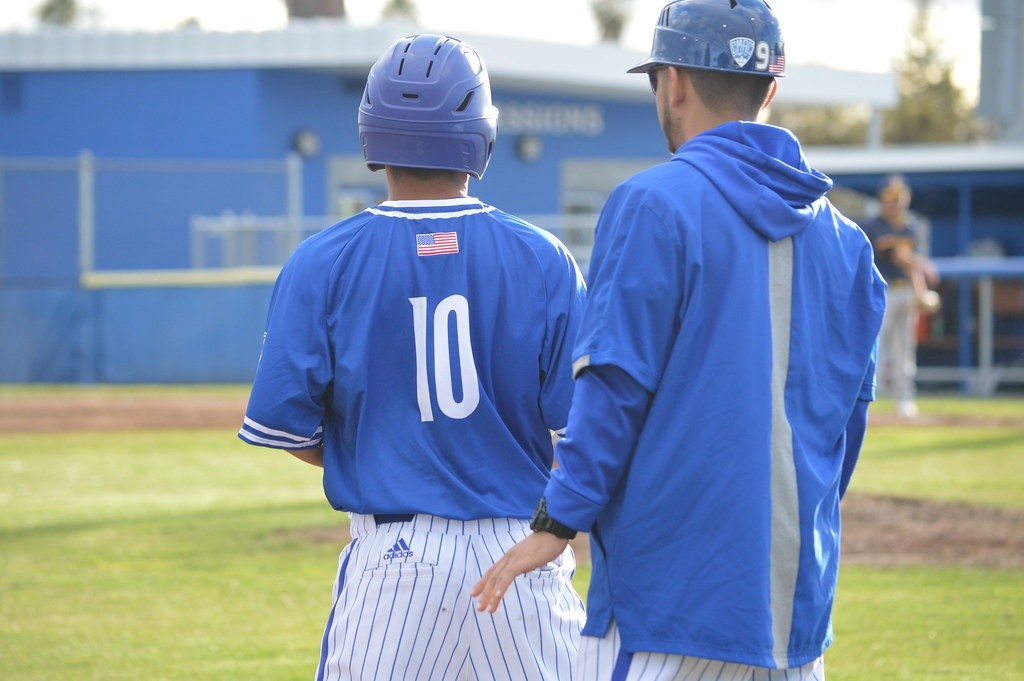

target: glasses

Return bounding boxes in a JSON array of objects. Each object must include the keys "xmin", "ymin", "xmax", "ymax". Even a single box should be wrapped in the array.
[{"xmin": 647, "ymin": 64, "xmax": 671, "ymax": 96}]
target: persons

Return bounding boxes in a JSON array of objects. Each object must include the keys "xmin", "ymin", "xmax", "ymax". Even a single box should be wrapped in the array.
[
  {"xmin": 235, "ymin": 32, "xmax": 586, "ymax": 680},
  {"xmin": 859, "ymin": 173, "xmax": 940, "ymax": 415},
  {"xmin": 470, "ymin": 0, "xmax": 887, "ymax": 681}
]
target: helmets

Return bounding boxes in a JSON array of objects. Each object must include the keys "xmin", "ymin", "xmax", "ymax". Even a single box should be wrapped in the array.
[
  {"xmin": 358, "ymin": 33, "xmax": 499, "ymax": 183},
  {"xmin": 626, "ymin": 0, "xmax": 787, "ymax": 79}
]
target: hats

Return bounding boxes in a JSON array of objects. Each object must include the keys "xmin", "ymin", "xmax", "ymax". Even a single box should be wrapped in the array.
[{"xmin": 877, "ymin": 173, "xmax": 913, "ymax": 203}]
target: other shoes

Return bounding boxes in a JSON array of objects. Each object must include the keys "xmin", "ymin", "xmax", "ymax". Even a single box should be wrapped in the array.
[{"xmin": 897, "ymin": 398, "xmax": 919, "ymax": 419}]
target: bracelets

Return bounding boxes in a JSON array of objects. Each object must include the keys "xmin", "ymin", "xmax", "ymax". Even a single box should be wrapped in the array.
[{"xmin": 530, "ymin": 496, "xmax": 577, "ymax": 540}]
